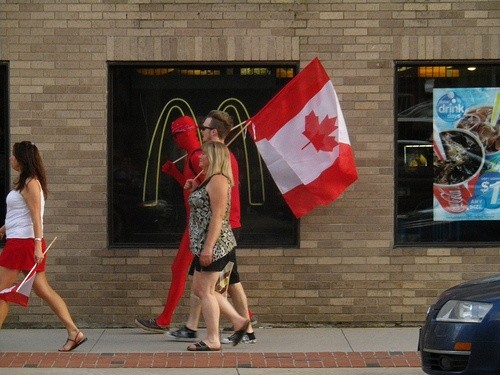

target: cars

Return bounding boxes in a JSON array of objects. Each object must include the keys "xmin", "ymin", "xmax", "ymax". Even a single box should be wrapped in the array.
[{"xmin": 416, "ymin": 273, "xmax": 500, "ymax": 375}]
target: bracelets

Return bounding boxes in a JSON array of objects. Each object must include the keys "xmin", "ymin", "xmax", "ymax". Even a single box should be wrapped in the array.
[{"xmin": 34, "ymin": 238, "xmax": 41, "ymax": 240}]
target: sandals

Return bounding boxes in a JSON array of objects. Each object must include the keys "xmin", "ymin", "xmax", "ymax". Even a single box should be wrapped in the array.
[{"xmin": 58, "ymin": 330, "xmax": 87, "ymax": 351}]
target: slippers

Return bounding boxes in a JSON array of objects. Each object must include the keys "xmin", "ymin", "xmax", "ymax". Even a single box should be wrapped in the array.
[
  {"xmin": 187, "ymin": 340, "xmax": 221, "ymax": 350},
  {"xmin": 231, "ymin": 319, "xmax": 250, "ymax": 346}
]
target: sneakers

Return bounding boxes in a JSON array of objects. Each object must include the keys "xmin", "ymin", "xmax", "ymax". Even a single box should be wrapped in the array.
[
  {"xmin": 134, "ymin": 318, "xmax": 170, "ymax": 333},
  {"xmin": 221, "ymin": 331, "xmax": 256, "ymax": 344},
  {"xmin": 222, "ymin": 326, "xmax": 235, "ymax": 333},
  {"xmin": 165, "ymin": 325, "xmax": 198, "ymax": 341}
]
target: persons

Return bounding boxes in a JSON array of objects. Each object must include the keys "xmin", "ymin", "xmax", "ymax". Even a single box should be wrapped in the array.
[
  {"xmin": 0, "ymin": 141, "xmax": 87, "ymax": 352},
  {"xmin": 183, "ymin": 142, "xmax": 251, "ymax": 352},
  {"xmin": 164, "ymin": 109, "xmax": 257, "ymax": 344},
  {"xmin": 134, "ymin": 116, "xmax": 256, "ymax": 333}
]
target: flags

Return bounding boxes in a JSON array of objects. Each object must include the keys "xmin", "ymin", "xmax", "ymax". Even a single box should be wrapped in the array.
[
  {"xmin": 0, "ymin": 264, "xmax": 38, "ymax": 306},
  {"xmin": 246, "ymin": 56, "xmax": 358, "ymax": 218}
]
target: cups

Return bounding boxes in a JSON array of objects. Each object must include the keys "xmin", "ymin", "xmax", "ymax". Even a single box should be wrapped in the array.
[{"xmin": 432, "ymin": 105, "xmax": 500, "ymax": 213}]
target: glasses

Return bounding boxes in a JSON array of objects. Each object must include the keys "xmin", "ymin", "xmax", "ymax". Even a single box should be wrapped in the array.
[{"xmin": 199, "ymin": 123, "xmax": 218, "ymax": 131}]
